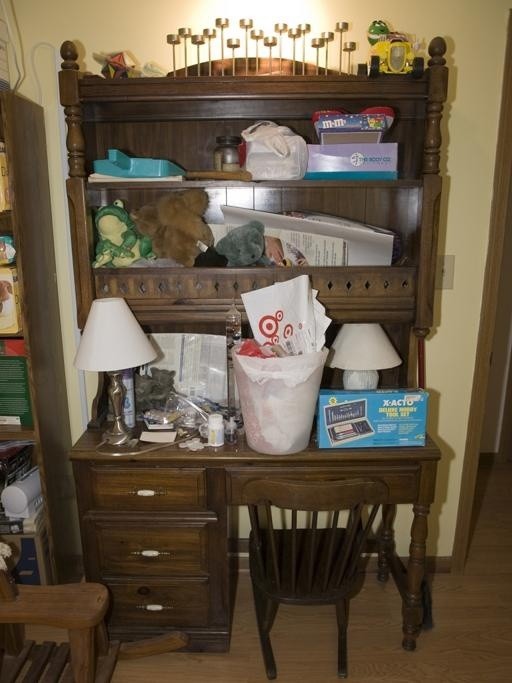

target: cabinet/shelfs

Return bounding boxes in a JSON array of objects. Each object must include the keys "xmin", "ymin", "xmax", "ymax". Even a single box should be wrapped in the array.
[
  {"xmin": 58, "ymin": 35, "xmax": 447, "ymax": 439},
  {"xmin": 0, "ymin": 79, "xmax": 83, "ymax": 585}
]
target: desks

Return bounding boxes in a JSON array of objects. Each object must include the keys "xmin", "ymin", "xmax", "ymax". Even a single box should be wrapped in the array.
[{"xmin": 65, "ymin": 418, "xmax": 442, "ymax": 654}]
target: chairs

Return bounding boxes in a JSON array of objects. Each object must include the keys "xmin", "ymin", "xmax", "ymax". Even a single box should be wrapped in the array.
[{"xmin": 242, "ymin": 479, "xmax": 386, "ymax": 680}]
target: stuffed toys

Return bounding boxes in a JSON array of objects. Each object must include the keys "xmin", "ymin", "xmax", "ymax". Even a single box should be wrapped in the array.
[{"xmin": 92, "ymin": 189, "xmax": 277, "ymax": 268}]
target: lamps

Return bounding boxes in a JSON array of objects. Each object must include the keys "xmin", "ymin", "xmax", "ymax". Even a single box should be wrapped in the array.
[
  {"xmin": 74, "ymin": 295, "xmax": 157, "ymax": 446},
  {"xmin": 326, "ymin": 320, "xmax": 404, "ymax": 391}
]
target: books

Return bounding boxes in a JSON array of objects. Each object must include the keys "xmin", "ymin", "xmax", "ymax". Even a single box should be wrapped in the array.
[
  {"xmin": 144, "ymin": 419, "xmax": 174, "ymax": 430},
  {"xmin": 139, "ymin": 421, "xmax": 178, "ymax": 443}
]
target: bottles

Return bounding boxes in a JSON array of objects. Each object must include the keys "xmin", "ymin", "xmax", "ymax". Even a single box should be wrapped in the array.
[
  {"xmin": 212, "ymin": 134, "xmax": 244, "ymax": 172},
  {"xmin": 207, "ymin": 413, "xmax": 225, "ymax": 447},
  {"xmin": 122, "ymin": 367, "xmax": 136, "ymax": 429},
  {"xmin": 226, "ymin": 416, "xmax": 237, "ymax": 444}
]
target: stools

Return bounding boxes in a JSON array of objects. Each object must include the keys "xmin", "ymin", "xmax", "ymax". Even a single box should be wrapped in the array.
[{"xmin": 0, "ymin": 568, "xmax": 189, "ymax": 683}]
